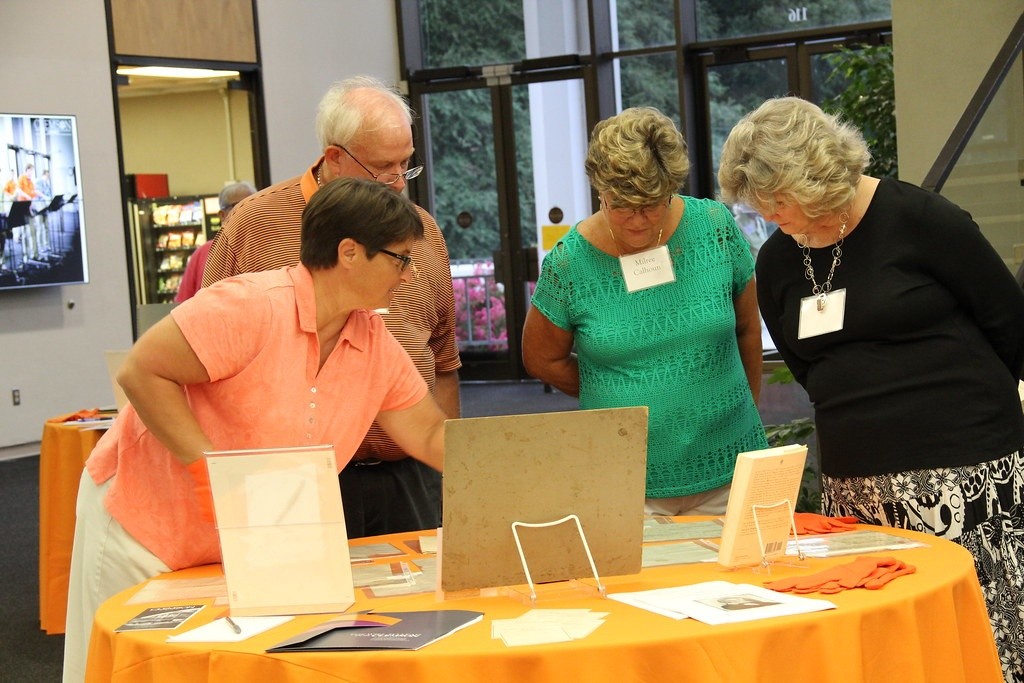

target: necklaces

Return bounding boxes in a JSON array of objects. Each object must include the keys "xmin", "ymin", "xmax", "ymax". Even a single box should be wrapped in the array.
[
  {"xmin": 609, "ymin": 228, "xmax": 663, "ymax": 257},
  {"xmin": 797, "ymin": 220, "xmax": 847, "ymax": 296}
]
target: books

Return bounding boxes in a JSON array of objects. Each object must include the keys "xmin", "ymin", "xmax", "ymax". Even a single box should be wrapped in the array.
[{"xmin": 719, "ymin": 444, "xmax": 808, "ymax": 567}]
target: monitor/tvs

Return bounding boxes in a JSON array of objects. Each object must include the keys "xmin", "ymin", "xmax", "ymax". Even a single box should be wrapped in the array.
[{"xmin": 0, "ymin": 112, "xmax": 90, "ymax": 290}]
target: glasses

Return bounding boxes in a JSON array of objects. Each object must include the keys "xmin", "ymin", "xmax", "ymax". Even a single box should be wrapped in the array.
[
  {"xmin": 333, "ymin": 143, "xmax": 425, "ymax": 185},
  {"xmin": 220, "ymin": 201, "xmax": 239, "ymax": 210},
  {"xmin": 357, "ymin": 240, "xmax": 412, "ymax": 272},
  {"xmin": 602, "ymin": 194, "xmax": 673, "ymax": 218}
]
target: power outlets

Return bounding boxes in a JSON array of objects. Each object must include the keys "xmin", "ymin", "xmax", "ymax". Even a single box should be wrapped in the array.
[{"xmin": 13, "ymin": 389, "xmax": 21, "ymax": 405}]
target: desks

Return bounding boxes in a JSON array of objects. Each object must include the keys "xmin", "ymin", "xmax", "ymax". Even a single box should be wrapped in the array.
[
  {"xmin": 38, "ymin": 407, "xmax": 116, "ymax": 635},
  {"xmin": 85, "ymin": 513, "xmax": 1002, "ymax": 683}
]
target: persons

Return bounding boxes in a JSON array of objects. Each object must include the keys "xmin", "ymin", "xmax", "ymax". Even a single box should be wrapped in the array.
[
  {"xmin": 0, "ymin": 163, "xmax": 52, "ymax": 274},
  {"xmin": 62, "ymin": 174, "xmax": 450, "ymax": 683},
  {"xmin": 201, "ymin": 76, "xmax": 462, "ymax": 540},
  {"xmin": 521, "ymin": 106, "xmax": 762, "ymax": 516},
  {"xmin": 717, "ymin": 596, "xmax": 779, "ymax": 610},
  {"xmin": 718, "ymin": 96, "xmax": 1024, "ymax": 683},
  {"xmin": 175, "ymin": 182, "xmax": 255, "ymax": 303}
]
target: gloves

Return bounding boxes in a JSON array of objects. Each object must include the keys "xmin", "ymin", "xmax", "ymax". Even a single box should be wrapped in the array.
[
  {"xmin": 762, "ymin": 556, "xmax": 916, "ymax": 595},
  {"xmin": 790, "ymin": 512, "xmax": 860, "ymax": 537}
]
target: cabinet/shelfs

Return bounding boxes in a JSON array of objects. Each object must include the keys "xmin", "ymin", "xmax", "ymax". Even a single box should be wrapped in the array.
[{"xmin": 134, "ymin": 195, "xmax": 222, "ymax": 305}]
